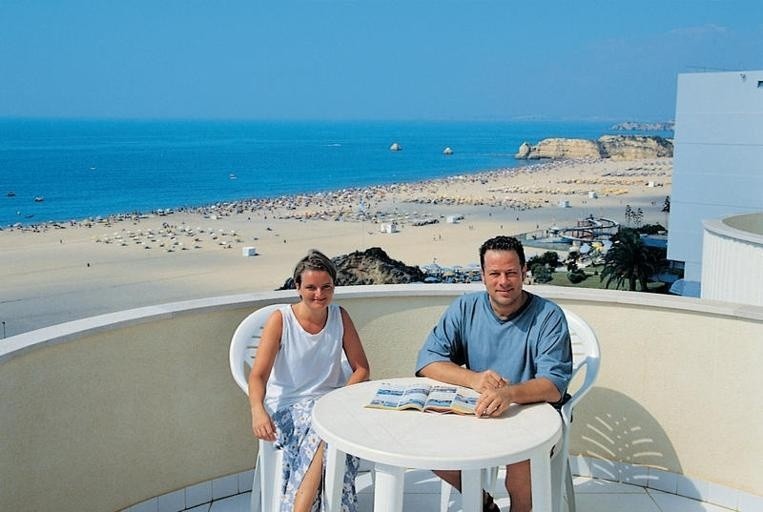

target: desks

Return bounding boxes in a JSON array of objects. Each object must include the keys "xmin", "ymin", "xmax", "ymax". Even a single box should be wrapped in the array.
[{"xmin": 311, "ymin": 371, "xmax": 567, "ymax": 512}]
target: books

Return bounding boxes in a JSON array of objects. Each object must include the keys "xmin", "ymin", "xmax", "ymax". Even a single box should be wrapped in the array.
[{"xmin": 364, "ymin": 379, "xmax": 493, "ymax": 419}]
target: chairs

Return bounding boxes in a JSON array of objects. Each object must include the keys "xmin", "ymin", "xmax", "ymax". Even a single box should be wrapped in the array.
[
  {"xmin": 437, "ymin": 299, "xmax": 603, "ymax": 512},
  {"xmin": 225, "ymin": 301, "xmax": 291, "ymax": 511}
]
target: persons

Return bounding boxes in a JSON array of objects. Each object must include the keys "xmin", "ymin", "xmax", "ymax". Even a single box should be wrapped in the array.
[
  {"xmin": 414, "ymin": 235, "xmax": 575, "ymax": 511},
  {"xmin": 247, "ymin": 248, "xmax": 371, "ymax": 511}
]
